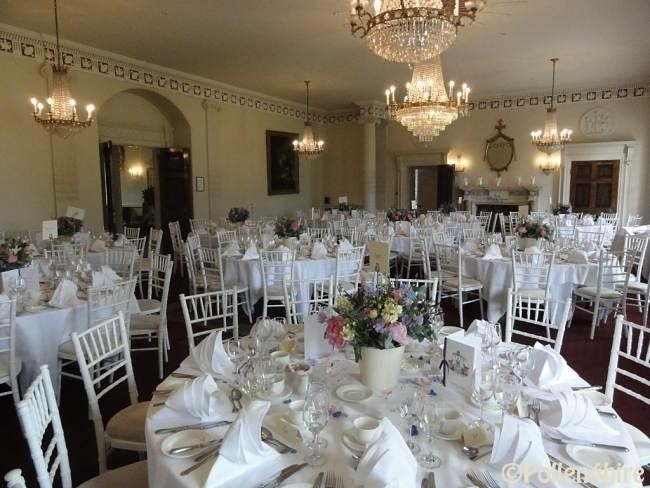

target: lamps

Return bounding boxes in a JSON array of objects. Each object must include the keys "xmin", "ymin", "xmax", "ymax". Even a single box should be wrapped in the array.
[
  {"xmin": 530, "ymin": 58, "xmax": 573, "ymax": 176},
  {"xmin": 446, "ymin": 147, "xmax": 472, "ymax": 172},
  {"xmin": 30, "ymin": 0, "xmax": 97, "ymax": 141},
  {"xmin": 386, "ymin": 57, "xmax": 473, "ymax": 149},
  {"xmin": 293, "ymin": 81, "xmax": 325, "ymax": 164},
  {"xmin": 349, "ymin": 0, "xmax": 484, "ymax": 65}
]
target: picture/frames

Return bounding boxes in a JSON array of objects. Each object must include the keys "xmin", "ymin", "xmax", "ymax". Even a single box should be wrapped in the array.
[{"xmin": 265, "ymin": 130, "xmax": 299, "ymax": 196}]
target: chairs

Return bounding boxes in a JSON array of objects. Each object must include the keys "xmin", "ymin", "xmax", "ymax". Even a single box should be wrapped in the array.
[{"xmin": 0, "ymin": 208, "xmax": 650, "ymax": 488}]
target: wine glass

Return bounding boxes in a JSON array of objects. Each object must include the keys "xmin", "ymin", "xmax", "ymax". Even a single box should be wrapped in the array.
[
  {"xmin": 7, "ymin": 259, "xmax": 96, "ymax": 309},
  {"xmin": 226, "ymin": 236, "xmax": 349, "ymax": 258},
  {"xmin": 469, "ymin": 233, "xmax": 589, "ymax": 264}
]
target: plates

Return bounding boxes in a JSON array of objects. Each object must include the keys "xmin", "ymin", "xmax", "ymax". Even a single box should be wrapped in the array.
[{"xmin": 150, "ymin": 316, "xmax": 630, "ymax": 487}]
